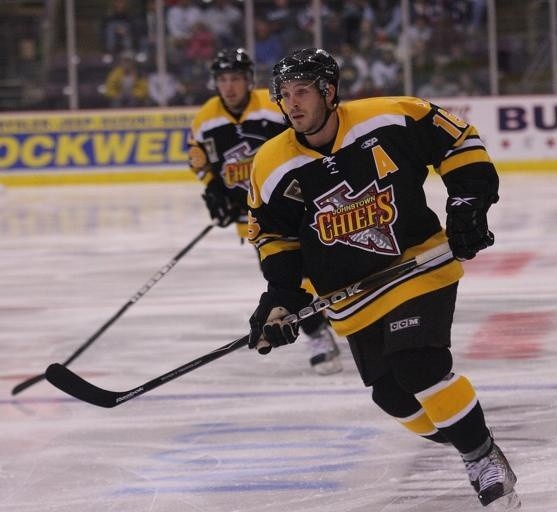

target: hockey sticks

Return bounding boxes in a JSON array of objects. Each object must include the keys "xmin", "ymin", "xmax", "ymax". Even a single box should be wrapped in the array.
[
  {"xmin": 46, "ymin": 229, "xmax": 494, "ymax": 408},
  {"xmin": 12, "ymin": 215, "xmax": 220, "ymax": 395}
]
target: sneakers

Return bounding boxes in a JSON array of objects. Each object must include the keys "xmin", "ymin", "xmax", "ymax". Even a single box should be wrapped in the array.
[
  {"xmin": 462, "ymin": 444, "xmax": 516, "ymax": 505},
  {"xmin": 307, "ymin": 331, "xmax": 338, "ymax": 363}
]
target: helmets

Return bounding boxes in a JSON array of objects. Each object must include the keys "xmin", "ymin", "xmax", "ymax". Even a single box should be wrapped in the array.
[
  {"xmin": 212, "ymin": 49, "xmax": 255, "ymax": 93},
  {"xmin": 272, "ymin": 48, "xmax": 340, "ymax": 102}
]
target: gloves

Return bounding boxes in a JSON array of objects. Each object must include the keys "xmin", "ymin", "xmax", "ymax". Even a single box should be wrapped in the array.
[
  {"xmin": 203, "ymin": 181, "xmax": 246, "ymax": 227},
  {"xmin": 247, "ymin": 287, "xmax": 313, "ymax": 354},
  {"xmin": 446, "ymin": 194, "xmax": 494, "ymax": 261}
]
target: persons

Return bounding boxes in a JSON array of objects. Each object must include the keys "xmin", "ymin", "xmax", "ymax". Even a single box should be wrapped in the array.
[
  {"xmin": 18, "ymin": 0, "xmax": 524, "ymax": 109},
  {"xmin": 246, "ymin": 46, "xmax": 517, "ymax": 506},
  {"xmin": 185, "ymin": 45, "xmax": 341, "ymax": 366}
]
